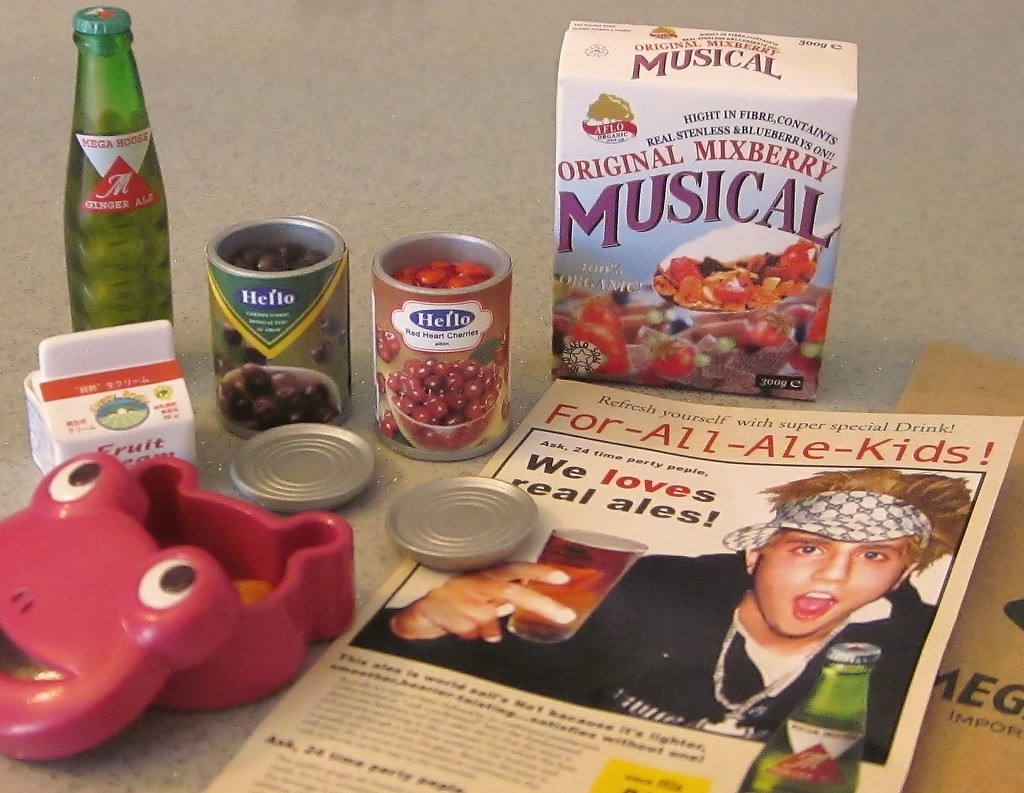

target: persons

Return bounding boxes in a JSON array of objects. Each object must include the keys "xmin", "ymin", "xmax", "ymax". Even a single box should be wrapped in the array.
[{"xmin": 356, "ymin": 468, "xmax": 974, "ymax": 765}]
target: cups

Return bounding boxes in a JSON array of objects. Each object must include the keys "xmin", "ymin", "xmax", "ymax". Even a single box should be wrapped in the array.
[{"xmin": 506, "ymin": 526, "xmax": 648, "ymax": 644}]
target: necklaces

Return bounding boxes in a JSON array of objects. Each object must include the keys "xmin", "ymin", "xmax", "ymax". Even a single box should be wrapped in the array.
[{"xmin": 696, "ymin": 589, "xmax": 852, "ymax": 740}]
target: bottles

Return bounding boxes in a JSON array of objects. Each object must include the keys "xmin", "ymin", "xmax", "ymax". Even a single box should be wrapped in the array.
[
  {"xmin": 737, "ymin": 641, "xmax": 883, "ymax": 793},
  {"xmin": 63, "ymin": 5, "xmax": 177, "ymax": 335}
]
